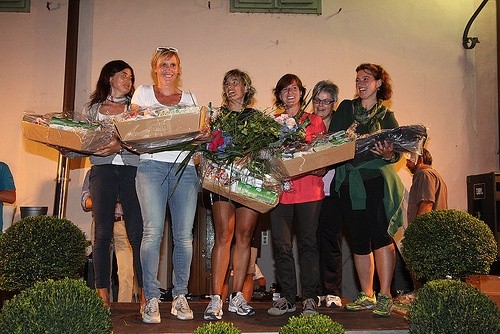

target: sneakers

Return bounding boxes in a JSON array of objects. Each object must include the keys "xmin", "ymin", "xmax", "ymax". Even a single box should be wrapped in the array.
[
  {"xmin": 302, "ymin": 298, "xmax": 320, "ymax": 315},
  {"xmin": 171, "ymin": 293, "xmax": 194, "ymax": 320},
  {"xmin": 373, "ymin": 293, "xmax": 395, "ymax": 317},
  {"xmin": 229, "ymin": 291, "xmax": 255, "ymax": 316},
  {"xmin": 204, "ymin": 295, "xmax": 223, "ymax": 321},
  {"xmin": 142, "ymin": 297, "xmax": 162, "ymax": 324},
  {"xmin": 345, "ymin": 292, "xmax": 376, "ymax": 311},
  {"xmin": 267, "ymin": 298, "xmax": 296, "ymax": 315}
]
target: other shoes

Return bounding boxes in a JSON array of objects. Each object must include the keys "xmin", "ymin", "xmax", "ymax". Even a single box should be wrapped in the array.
[{"xmin": 324, "ymin": 294, "xmax": 342, "ymax": 308}]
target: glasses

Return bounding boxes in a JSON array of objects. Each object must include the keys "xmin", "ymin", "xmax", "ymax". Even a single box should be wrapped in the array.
[
  {"xmin": 312, "ymin": 99, "xmax": 335, "ymax": 105},
  {"xmin": 154, "ymin": 47, "xmax": 178, "ymax": 54}
]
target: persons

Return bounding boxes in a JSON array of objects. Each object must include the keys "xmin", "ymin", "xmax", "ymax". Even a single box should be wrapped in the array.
[
  {"xmin": 205, "ymin": 70, "xmax": 258, "ymax": 320},
  {"xmin": 327, "ymin": 64, "xmax": 405, "ymax": 317},
  {"xmin": 222, "ymin": 230, "xmax": 261, "ymax": 304},
  {"xmin": 0, "ymin": 162, "xmax": 16, "ymax": 233},
  {"xmin": 312, "ymin": 80, "xmax": 343, "ymax": 306},
  {"xmin": 49, "ymin": 60, "xmax": 147, "ymax": 312},
  {"xmin": 131, "ymin": 48, "xmax": 199, "ymax": 322},
  {"xmin": 268, "ymin": 73, "xmax": 327, "ymax": 314},
  {"xmin": 81, "ymin": 169, "xmax": 134, "ymax": 303},
  {"xmin": 406, "ymin": 148, "xmax": 447, "ymax": 225}
]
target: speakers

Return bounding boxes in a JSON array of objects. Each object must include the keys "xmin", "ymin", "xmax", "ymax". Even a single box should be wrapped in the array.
[{"xmin": 466, "ymin": 172, "xmax": 500, "ymax": 259}]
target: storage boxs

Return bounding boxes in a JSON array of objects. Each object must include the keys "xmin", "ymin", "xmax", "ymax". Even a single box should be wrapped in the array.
[
  {"xmin": 199, "ymin": 169, "xmax": 282, "ymax": 216},
  {"xmin": 18, "ymin": 111, "xmax": 97, "ymax": 155},
  {"xmin": 110, "ymin": 105, "xmax": 210, "ymax": 154},
  {"xmin": 274, "ymin": 140, "xmax": 360, "ymax": 179}
]
